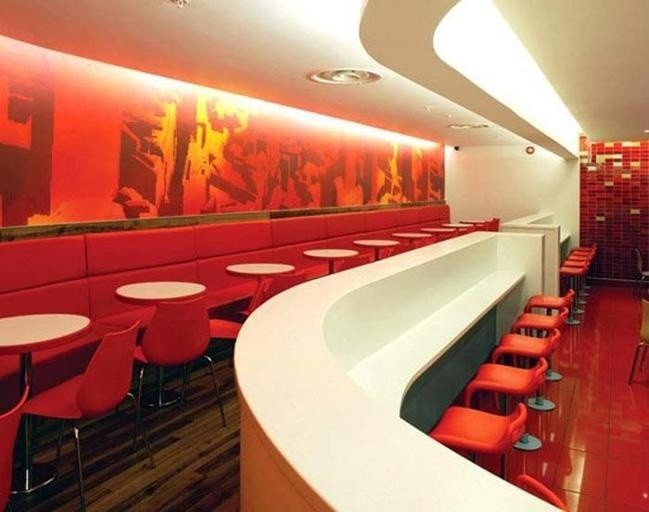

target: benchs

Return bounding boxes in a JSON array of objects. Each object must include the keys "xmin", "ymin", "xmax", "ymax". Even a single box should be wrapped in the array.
[{"xmin": 0, "ymin": 203, "xmax": 453, "ymax": 386}]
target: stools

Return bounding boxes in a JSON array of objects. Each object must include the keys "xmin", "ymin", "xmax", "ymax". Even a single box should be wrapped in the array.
[
  {"xmin": 508, "ymin": 305, "xmax": 569, "ymax": 412},
  {"xmin": 520, "ymin": 287, "xmax": 574, "ymax": 382},
  {"xmin": 427, "ymin": 401, "xmax": 530, "ymax": 464},
  {"xmin": 516, "ymin": 472, "xmax": 568, "ymax": 510},
  {"xmin": 489, "ymin": 326, "xmax": 561, "ymax": 452},
  {"xmin": 461, "ymin": 354, "xmax": 549, "ymax": 481},
  {"xmin": 560, "ymin": 240, "xmax": 599, "ymax": 328}
]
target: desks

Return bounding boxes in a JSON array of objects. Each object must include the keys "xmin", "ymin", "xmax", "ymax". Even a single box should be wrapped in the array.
[
  {"xmin": 116, "ymin": 280, "xmax": 207, "ymax": 409},
  {"xmin": 223, "ymin": 220, "xmax": 488, "ymax": 317},
  {"xmin": 1, "ymin": 309, "xmax": 93, "ymax": 498}
]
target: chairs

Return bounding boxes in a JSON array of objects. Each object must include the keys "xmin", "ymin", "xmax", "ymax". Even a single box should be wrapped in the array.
[
  {"xmin": 21, "ymin": 320, "xmax": 155, "ymax": 512},
  {"xmin": 630, "ymin": 247, "xmax": 649, "ymax": 296},
  {"xmin": 628, "ymin": 299, "xmax": 649, "ymax": 384},
  {"xmin": 111, "ymin": 295, "xmax": 230, "ymax": 452},
  {"xmin": 1, "ymin": 385, "xmax": 30, "ymax": 512}
]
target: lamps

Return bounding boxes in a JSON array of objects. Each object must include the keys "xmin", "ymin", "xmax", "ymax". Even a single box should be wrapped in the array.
[{"xmin": 580, "ymin": 136, "xmax": 590, "ymax": 166}]
